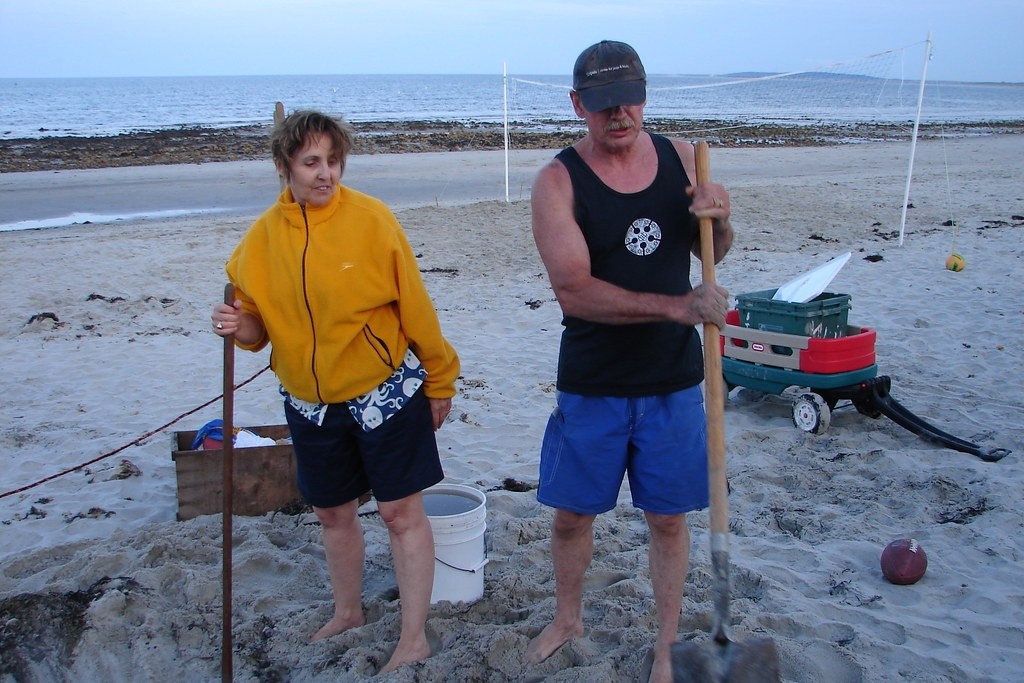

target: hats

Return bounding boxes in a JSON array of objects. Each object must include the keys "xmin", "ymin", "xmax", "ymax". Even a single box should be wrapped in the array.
[{"xmin": 573, "ymin": 40, "xmax": 647, "ymax": 113}]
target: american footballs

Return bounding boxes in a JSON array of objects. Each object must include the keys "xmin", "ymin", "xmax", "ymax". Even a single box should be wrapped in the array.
[{"xmin": 880, "ymin": 538, "xmax": 928, "ymax": 584}]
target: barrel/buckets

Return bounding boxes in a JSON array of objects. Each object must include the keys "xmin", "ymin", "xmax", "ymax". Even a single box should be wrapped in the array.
[{"xmin": 422, "ymin": 483, "xmax": 489, "ymax": 606}]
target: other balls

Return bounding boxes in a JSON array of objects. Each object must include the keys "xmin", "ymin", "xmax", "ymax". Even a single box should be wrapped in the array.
[{"xmin": 945, "ymin": 254, "xmax": 964, "ymax": 272}]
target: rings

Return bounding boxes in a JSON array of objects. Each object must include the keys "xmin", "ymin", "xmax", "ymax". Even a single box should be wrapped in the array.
[
  {"xmin": 446, "ymin": 410, "xmax": 451, "ymax": 416},
  {"xmin": 714, "ymin": 199, "xmax": 723, "ymax": 208},
  {"xmin": 216, "ymin": 320, "xmax": 223, "ymax": 329}
]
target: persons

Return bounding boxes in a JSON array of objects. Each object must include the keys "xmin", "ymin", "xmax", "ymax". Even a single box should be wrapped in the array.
[
  {"xmin": 211, "ymin": 111, "xmax": 461, "ymax": 679},
  {"xmin": 530, "ymin": 40, "xmax": 733, "ymax": 683}
]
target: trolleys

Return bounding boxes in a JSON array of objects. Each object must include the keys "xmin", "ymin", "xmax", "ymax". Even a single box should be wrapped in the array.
[{"xmin": 709, "ymin": 288, "xmax": 1015, "ymax": 461}]
